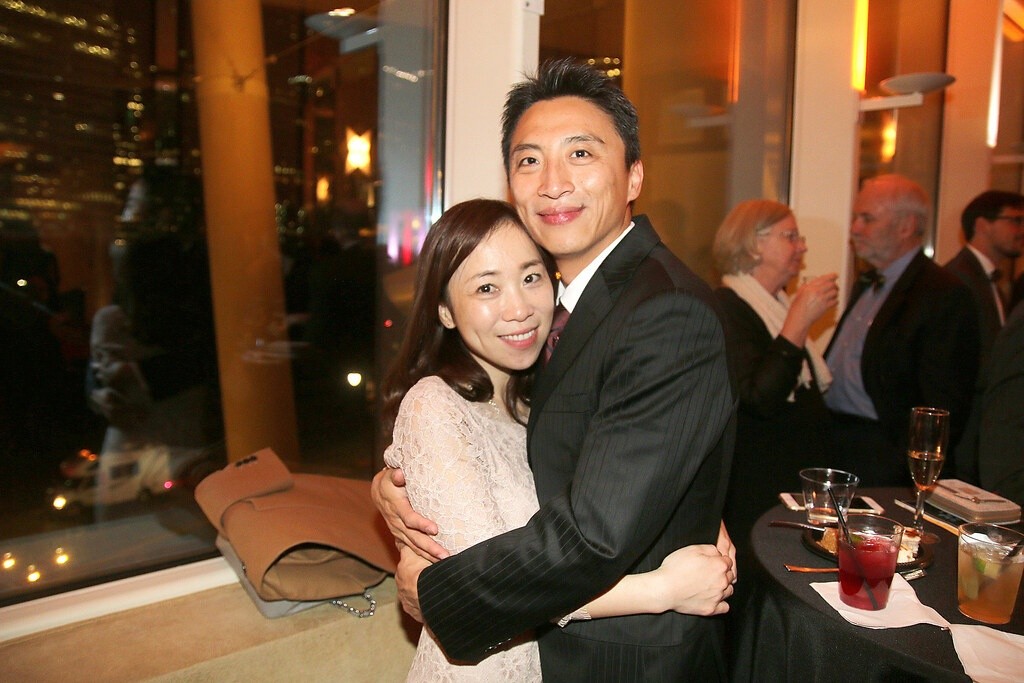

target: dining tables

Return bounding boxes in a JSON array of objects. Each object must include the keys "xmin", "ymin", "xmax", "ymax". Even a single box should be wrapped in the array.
[{"xmin": 724, "ymin": 486, "xmax": 1024, "ymax": 683}]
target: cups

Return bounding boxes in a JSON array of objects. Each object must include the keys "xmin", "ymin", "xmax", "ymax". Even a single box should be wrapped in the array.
[
  {"xmin": 957, "ymin": 521, "xmax": 1024, "ymax": 624},
  {"xmin": 800, "ymin": 467, "xmax": 860, "ymax": 525},
  {"xmin": 838, "ymin": 513, "xmax": 904, "ymax": 611},
  {"xmin": 803, "ymin": 276, "xmax": 818, "ymax": 285}
]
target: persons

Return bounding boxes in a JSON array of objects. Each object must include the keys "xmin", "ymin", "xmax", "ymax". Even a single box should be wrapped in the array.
[
  {"xmin": 713, "ymin": 197, "xmax": 839, "ymax": 577},
  {"xmin": 1, "ymin": 161, "xmax": 228, "ymax": 524},
  {"xmin": 821, "ymin": 173, "xmax": 977, "ymax": 493},
  {"xmin": 939, "ymin": 184, "xmax": 1024, "ymax": 490},
  {"xmin": 371, "ymin": 60, "xmax": 737, "ymax": 683}
]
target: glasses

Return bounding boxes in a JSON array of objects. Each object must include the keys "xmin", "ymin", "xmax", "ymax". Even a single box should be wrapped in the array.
[
  {"xmin": 782, "ymin": 231, "xmax": 806, "ymax": 242},
  {"xmin": 998, "ymin": 216, "xmax": 1024, "ymax": 225}
]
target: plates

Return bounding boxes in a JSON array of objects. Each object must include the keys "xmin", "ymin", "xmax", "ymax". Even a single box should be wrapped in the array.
[{"xmin": 802, "ymin": 523, "xmax": 933, "ymax": 569}]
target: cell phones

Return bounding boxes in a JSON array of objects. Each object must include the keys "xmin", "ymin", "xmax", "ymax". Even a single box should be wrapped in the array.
[{"xmin": 779, "ymin": 492, "xmax": 885, "ymax": 515}]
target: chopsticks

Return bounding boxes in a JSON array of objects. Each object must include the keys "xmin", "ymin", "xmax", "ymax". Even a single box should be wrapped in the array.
[{"xmin": 894, "ymin": 498, "xmax": 960, "ymax": 536}]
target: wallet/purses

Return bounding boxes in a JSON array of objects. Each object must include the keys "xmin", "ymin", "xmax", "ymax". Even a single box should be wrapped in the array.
[{"xmin": 917, "ymin": 479, "xmax": 1021, "ymax": 525}]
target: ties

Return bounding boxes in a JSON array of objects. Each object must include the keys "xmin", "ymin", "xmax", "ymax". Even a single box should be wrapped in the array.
[
  {"xmin": 990, "ymin": 270, "xmax": 1009, "ymax": 320},
  {"xmin": 542, "ymin": 302, "xmax": 571, "ymax": 371}
]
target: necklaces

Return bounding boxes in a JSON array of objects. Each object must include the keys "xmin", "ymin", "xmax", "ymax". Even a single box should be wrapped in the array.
[{"xmin": 488, "ymin": 400, "xmax": 501, "ymax": 414}]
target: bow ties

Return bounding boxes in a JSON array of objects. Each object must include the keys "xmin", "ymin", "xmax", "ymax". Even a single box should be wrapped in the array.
[{"xmin": 859, "ymin": 271, "xmax": 885, "ymax": 296}]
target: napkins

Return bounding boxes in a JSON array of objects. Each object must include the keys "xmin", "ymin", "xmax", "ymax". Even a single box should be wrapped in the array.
[
  {"xmin": 947, "ymin": 624, "xmax": 1024, "ymax": 683},
  {"xmin": 808, "ymin": 571, "xmax": 950, "ymax": 628}
]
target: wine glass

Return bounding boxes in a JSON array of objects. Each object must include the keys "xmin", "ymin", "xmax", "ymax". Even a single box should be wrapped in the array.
[{"xmin": 911, "ymin": 405, "xmax": 950, "ymax": 547}]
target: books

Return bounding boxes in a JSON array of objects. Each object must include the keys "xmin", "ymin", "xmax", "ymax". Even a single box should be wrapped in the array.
[{"xmin": 893, "ymin": 497, "xmax": 1024, "ymax": 540}]
